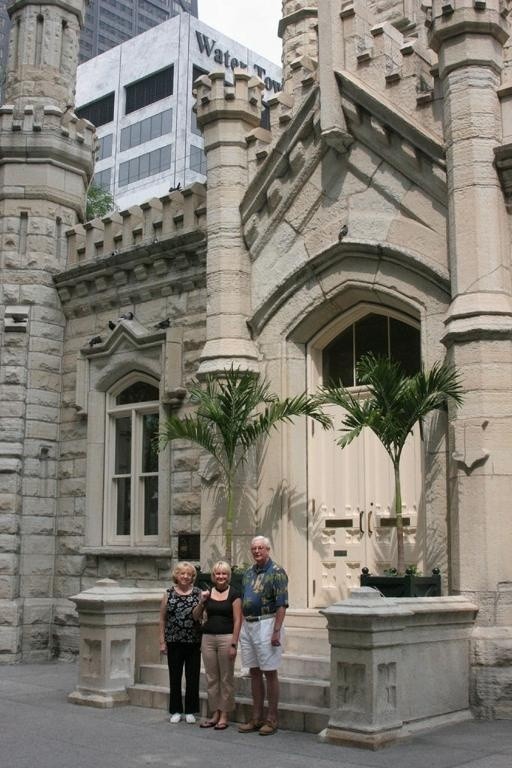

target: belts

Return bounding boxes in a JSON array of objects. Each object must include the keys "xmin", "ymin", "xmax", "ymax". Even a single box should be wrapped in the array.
[{"xmin": 246, "ymin": 618, "xmax": 259, "ymax": 622}]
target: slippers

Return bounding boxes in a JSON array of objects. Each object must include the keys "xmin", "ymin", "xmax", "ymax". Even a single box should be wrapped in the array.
[
  {"xmin": 214, "ymin": 723, "xmax": 228, "ymax": 730},
  {"xmin": 200, "ymin": 721, "xmax": 217, "ymax": 727}
]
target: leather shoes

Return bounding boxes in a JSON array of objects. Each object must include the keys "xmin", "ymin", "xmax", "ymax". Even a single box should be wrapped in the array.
[
  {"xmin": 259, "ymin": 722, "xmax": 278, "ymax": 734},
  {"xmin": 238, "ymin": 719, "xmax": 262, "ymax": 732}
]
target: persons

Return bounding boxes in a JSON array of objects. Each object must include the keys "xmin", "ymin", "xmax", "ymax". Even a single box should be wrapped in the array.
[
  {"xmin": 238, "ymin": 535, "xmax": 289, "ymax": 734},
  {"xmin": 192, "ymin": 560, "xmax": 243, "ymax": 730},
  {"xmin": 158, "ymin": 560, "xmax": 206, "ymax": 723}
]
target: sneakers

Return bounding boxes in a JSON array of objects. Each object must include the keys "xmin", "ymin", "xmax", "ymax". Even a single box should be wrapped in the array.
[
  {"xmin": 185, "ymin": 714, "xmax": 196, "ymax": 723},
  {"xmin": 170, "ymin": 713, "xmax": 181, "ymax": 723}
]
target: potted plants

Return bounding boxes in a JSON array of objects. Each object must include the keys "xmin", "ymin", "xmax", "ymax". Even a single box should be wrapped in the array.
[
  {"xmin": 148, "ymin": 356, "xmax": 335, "ymax": 598},
  {"xmin": 309, "ymin": 349, "xmax": 469, "ymax": 598}
]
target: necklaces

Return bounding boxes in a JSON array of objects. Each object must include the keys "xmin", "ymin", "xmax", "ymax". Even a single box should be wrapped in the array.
[{"xmin": 215, "ymin": 586, "xmax": 228, "ymax": 592}]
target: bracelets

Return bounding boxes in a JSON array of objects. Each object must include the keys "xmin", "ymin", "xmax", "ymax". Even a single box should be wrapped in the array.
[
  {"xmin": 199, "ymin": 599, "xmax": 204, "ymax": 607},
  {"xmin": 232, "ymin": 642, "xmax": 237, "ymax": 648},
  {"xmin": 274, "ymin": 629, "xmax": 280, "ymax": 631},
  {"xmin": 159, "ymin": 640, "xmax": 165, "ymax": 644}
]
